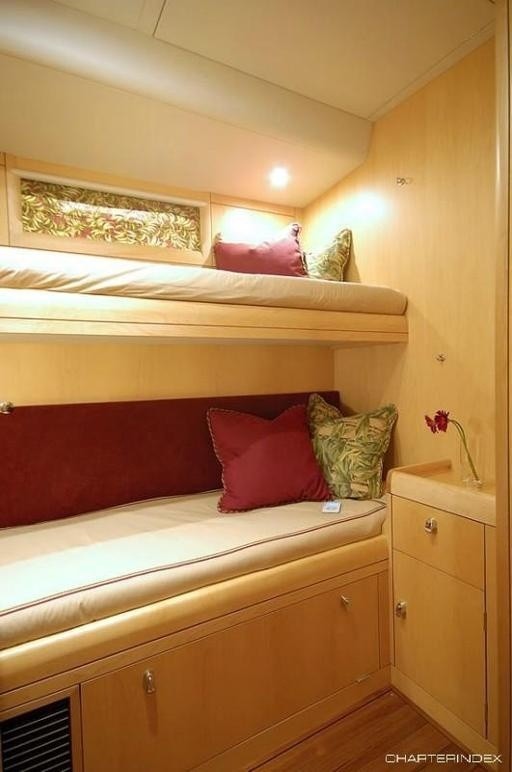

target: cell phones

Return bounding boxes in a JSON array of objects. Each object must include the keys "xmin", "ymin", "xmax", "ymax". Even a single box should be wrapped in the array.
[{"xmin": 322, "ymin": 502, "xmax": 341, "ymax": 514}]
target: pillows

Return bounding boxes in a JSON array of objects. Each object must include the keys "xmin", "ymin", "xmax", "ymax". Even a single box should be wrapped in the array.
[
  {"xmin": 206, "ymin": 406, "xmax": 328, "ymax": 506},
  {"xmin": 211, "ymin": 223, "xmax": 305, "ymax": 277},
  {"xmin": 309, "ymin": 394, "xmax": 399, "ymax": 498},
  {"xmin": 305, "ymin": 229, "xmax": 351, "ymax": 280}
]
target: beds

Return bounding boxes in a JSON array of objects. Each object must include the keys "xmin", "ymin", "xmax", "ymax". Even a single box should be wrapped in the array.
[{"xmin": 0, "ymin": 250, "xmax": 407, "ymax": 772}]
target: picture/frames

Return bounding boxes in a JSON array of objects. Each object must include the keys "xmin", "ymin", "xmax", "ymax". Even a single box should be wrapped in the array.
[{"xmin": 7, "ymin": 155, "xmax": 212, "ymax": 268}]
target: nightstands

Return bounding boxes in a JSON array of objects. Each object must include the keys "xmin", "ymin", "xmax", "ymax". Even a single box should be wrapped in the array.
[{"xmin": 388, "ymin": 462, "xmax": 512, "ymax": 771}]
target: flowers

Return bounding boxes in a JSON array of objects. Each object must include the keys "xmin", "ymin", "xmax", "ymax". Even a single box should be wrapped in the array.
[{"xmin": 424, "ymin": 411, "xmax": 479, "ymax": 481}]
